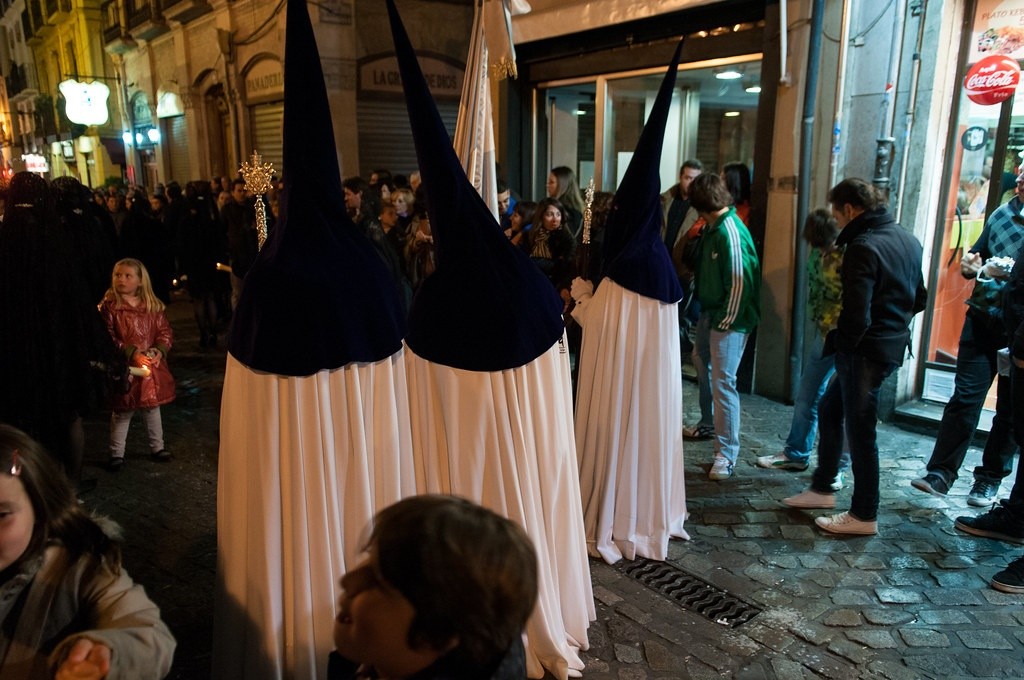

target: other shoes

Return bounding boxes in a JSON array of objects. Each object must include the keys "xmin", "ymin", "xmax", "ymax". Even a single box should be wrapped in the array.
[
  {"xmin": 151, "ymin": 450, "xmax": 172, "ymax": 460},
  {"xmin": 78, "ymin": 478, "xmax": 98, "ymax": 493},
  {"xmin": 109, "ymin": 457, "xmax": 126, "ymax": 469}
]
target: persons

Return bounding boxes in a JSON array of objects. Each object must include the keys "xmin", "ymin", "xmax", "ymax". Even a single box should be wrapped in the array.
[{"xmin": 0, "ymin": 149, "xmax": 1024, "ymax": 680}]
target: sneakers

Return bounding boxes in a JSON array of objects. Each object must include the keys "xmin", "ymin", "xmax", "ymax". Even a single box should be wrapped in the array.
[
  {"xmin": 782, "ymin": 490, "xmax": 836, "ymax": 508},
  {"xmin": 967, "ymin": 478, "xmax": 1001, "ymax": 507},
  {"xmin": 911, "ymin": 474, "xmax": 949, "ymax": 498},
  {"xmin": 709, "ymin": 453, "xmax": 733, "ymax": 479},
  {"xmin": 757, "ymin": 453, "xmax": 809, "ymax": 470},
  {"xmin": 814, "ymin": 512, "xmax": 878, "ymax": 534},
  {"xmin": 828, "ymin": 472, "xmax": 845, "ymax": 491},
  {"xmin": 682, "ymin": 421, "xmax": 716, "ymax": 437},
  {"xmin": 991, "ymin": 553, "xmax": 1024, "ymax": 593},
  {"xmin": 954, "ymin": 500, "xmax": 1024, "ymax": 544}
]
